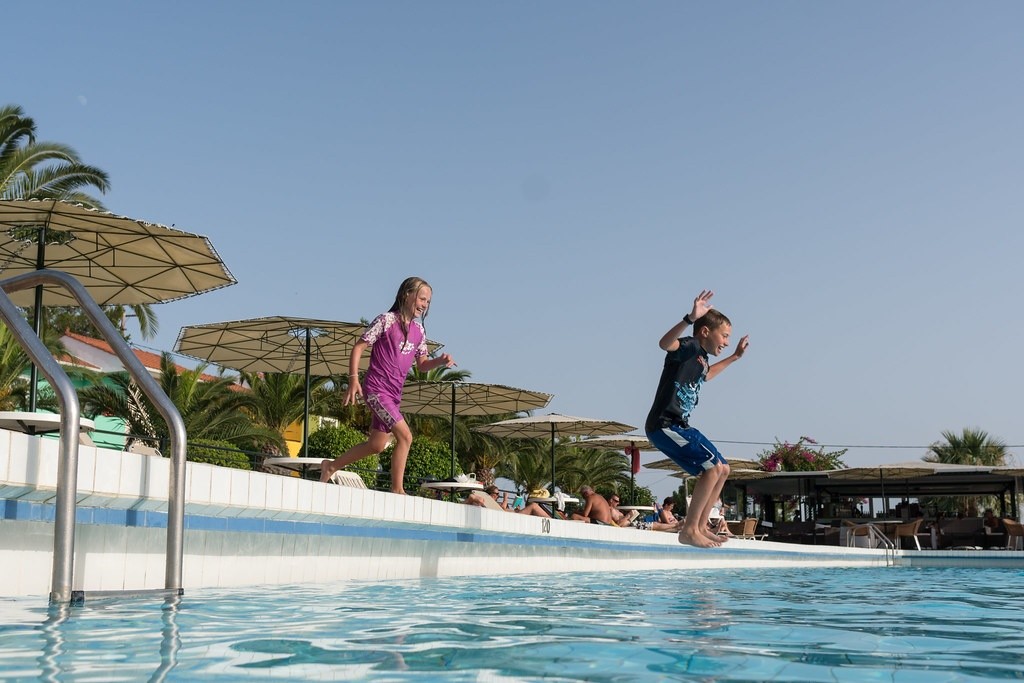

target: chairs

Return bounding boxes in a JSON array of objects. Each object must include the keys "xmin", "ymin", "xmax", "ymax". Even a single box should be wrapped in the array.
[
  {"xmin": 708, "ymin": 508, "xmax": 771, "ymax": 539},
  {"xmin": 893, "ymin": 517, "xmax": 925, "ymax": 550},
  {"xmin": 329, "ymin": 469, "xmax": 369, "ymax": 489},
  {"xmin": 735, "ymin": 518, "xmax": 758, "ymax": 540},
  {"xmin": 1002, "ymin": 518, "xmax": 1024, "ymax": 551},
  {"xmin": 844, "ymin": 517, "xmax": 877, "ymax": 548},
  {"xmin": 472, "ymin": 489, "xmax": 506, "ymax": 511}
]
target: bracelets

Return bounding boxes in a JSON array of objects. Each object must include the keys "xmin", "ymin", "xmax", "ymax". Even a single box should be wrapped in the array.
[{"xmin": 683, "ymin": 313, "xmax": 693, "ymax": 325}]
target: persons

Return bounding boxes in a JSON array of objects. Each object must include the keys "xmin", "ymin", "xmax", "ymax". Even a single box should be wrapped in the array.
[
  {"xmin": 644, "ymin": 289, "xmax": 750, "ymax": 547},
  {"xmin": 974, "ymin": 509, "xmax": 1004, "ymax": 533},
  {"xmin": 660, "ymin": 496, "xmax": 727, "ymax": 533},
  {"xmin": 793, "ymin": 509, "xmax": 801, "ymax": 523},
  {"xmin": 607, "ymin": 494, "xmax": 686, "ymax": 533},
  {"xmin": 318, "ymin": 276, "xmax": 458, "ymax": 496},
  {"xmin": 572, "ymin": 485, "xmax": 611, "ymax": 525},
  {"xmin": 462, "ymin": 494, "xmax": 485, "ymax": 506}
]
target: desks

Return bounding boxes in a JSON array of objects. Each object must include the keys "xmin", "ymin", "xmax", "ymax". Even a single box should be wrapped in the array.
[
  {"xmin": 528, "ymin": 496, "xmax": 579, "ymax": 519},
  {"xmin": 420, "ymin": 481, "xmax": 484, "ymax": 502},
  {"xmin": 864, "ymin": 519, "xmax": 903, "ymax": 549},
  {"xmin": 0, "ymin": 411, "xmax": 96, "ymax": 436},
  {"xmin": 616, "ymin": 505, "xmax": 654, "ymax": 528},
  {"xmin": 263, "ymin": 457, "xmax": 336, "ymax": 480},
  {"xmin": 941, "ymin": 529, "xmax": 984, "ymax": 550}
]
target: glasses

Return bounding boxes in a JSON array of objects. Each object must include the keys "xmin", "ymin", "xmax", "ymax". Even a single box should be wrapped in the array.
[
  {"xmin": 613, "ymin": 499, "xmax": 619, "ymax": 503},
  {"xmin": 491, "ymin": 491, "xmax": 499, "ymax": 495}
]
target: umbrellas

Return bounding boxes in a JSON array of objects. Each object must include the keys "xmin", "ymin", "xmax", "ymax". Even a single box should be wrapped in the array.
[
  {"xmin": 471, "ymin": 412, "xmax": 935, "ymax": 522},
  {"xmin": 172, "ymin": 314, "xmax": 445, "ymax": 458},
  {"xmin": 1, "ymin": 198, "xmax": 239, "ymax": 413},
  {"xmin": 398, "ymin": 378, "xmax": 554, "ymax": 478}
]
